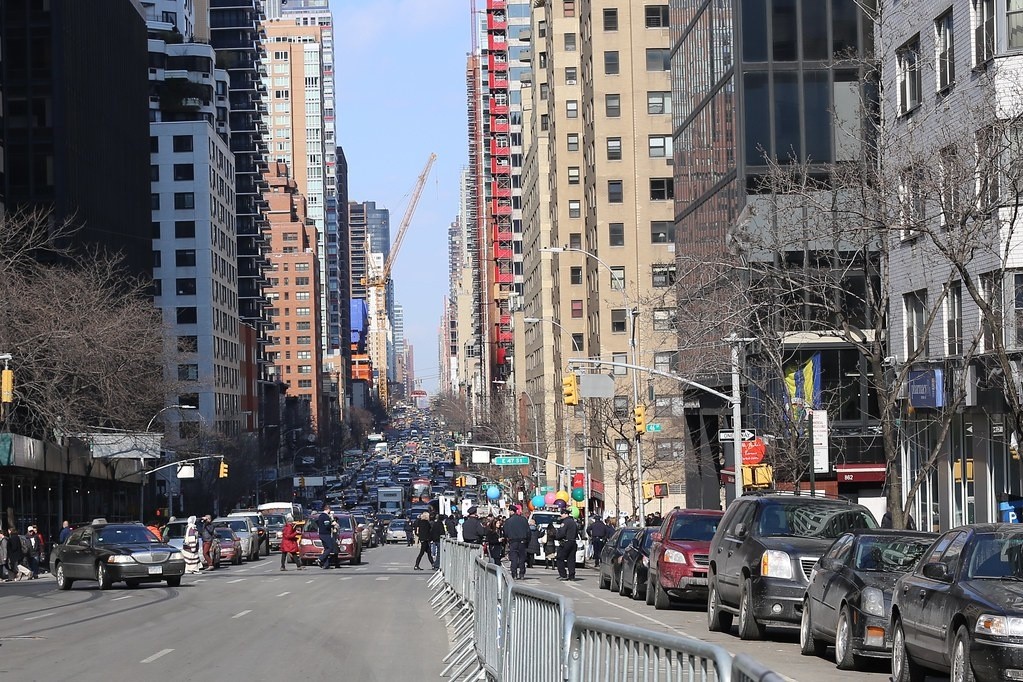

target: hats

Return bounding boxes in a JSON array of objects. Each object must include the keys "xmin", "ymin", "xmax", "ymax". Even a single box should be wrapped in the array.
[
  {"xmin": 594, "ymin": 516, "xmax": 601, "ymax": 521},
  {"xmin": 508, "ymin": 504, "xmax": 518, "ymax": 512},
  {"xmin": 468, "ymin": 506, "xmax": 477, "ymax": 514},
  {"xmin": 28, "ymin": 526, "xmax": 34, "ymax": 531},
  {"xmin": 561, "ymin": 508, "xmax": 571, "ymax": 515}
]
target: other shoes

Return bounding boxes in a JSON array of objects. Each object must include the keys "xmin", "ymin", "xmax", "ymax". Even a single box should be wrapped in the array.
[
  {"xmin": 280, "ymin": 567, "xmax": 284, "ymax": 571},
  {"xmin": 520, "ymin": 576, "xmax": 524, "ymax": 579},
  {"xmin": 335, "ymin": 565, "xmax": 342, "ymax": 568},
  {"xmin": 205, "ymin": 566, "xmax": 215, "ymax": 571},
  {"xmin": 324, "ymin": 565, "xmax": 333, "ymax": 569},
  {"xmin": 566, "ymin": 576, "xmax": 574, "ymax": 581},
  {"xmin": 17, "ymin": 573, "xmax": 23, "ymax": 581},
  {"xmin": 0, "ymin": 578, "xmax": 3, "ymax": 583},
  {"xmin": 297, "ymin": 567, "xmax": 306, "ymax": 570},
  {"xmin": 315, "ymin": 558, "xmax": 322, "ymax": 568},
  {"xmin": 194, "ymin": 571, "xmax": 202, "ymax": 575},
  {"xmin": 556, "ymin": 576, "xmax": 566, "ymax": 579},
  {"xmin": 5, "ymin": 579, "xmax": 15, "ymax": 583},
  {"xmin": 513, "ymin": 576, "xmax": 517, "ymax": 579},
  {"xmin": 34, "ymin": 575, "xmax": 38, "ymax": 579},
  {"xmin": 28, "ymin": 571, "xmax": 35, "ymax": 580},
  {"xmin": 414, "ymin": 566, "xmax": 423, "ymax": 570}
]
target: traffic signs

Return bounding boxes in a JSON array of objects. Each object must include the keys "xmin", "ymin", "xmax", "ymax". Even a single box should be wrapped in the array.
[{"xmin": 719, "ymin": 429, "xmax": 756, "ymax": 442}]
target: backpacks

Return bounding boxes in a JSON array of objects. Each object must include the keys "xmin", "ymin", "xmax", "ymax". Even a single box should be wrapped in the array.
[{"xmin": 16, "ymin": 535, "xmax": 30, "ymax": 554}]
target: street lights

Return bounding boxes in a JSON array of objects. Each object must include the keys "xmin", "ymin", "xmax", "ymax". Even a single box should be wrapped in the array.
[
  {"xmin": 254, "ymin": 423, "xmax": 316, "ymax": 506},
  {"xmin": 525, "ymin": 316, "xmax": 591, "ymax": 526},
  {"xmin": 138, "ymin": 406, "xmax": 197, "ymax": 523},
  {"xmin": 492, "ymin": 379, "xmax": 539, "ymax": 496},
  {"xmin": 540, "ymin": 246, "xmax": 654, "ymax": 526}
]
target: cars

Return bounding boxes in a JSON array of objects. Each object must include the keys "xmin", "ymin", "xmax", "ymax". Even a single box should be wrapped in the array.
[
  {"xmin": 51, "ymin": 516, "xmax": 185, "ymax": 592},
  {"xmin": 597, "ymin": 527, "xmax": 660, "ymax": 599},
  {"xmin": 798, "ymin": 528, "xmax": 941, "ymax": 670},
  {"xmin": 307, "ymin": 406, "xmax": 483, "ymax": 549},
  {"xmin": 890, "ymin": 522, "xmax": 1023, "ymax": 682},
  {"xmin": 214, "ymin": 528, "xmax": 243, "ymax": 565}
]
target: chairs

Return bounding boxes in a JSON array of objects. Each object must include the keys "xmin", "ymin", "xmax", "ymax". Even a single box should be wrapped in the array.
[
  {"xmin": 765, "ymin": 512, "xmax": 781, "ymax": 533},
  {"xmin": 623, "ymin": 539, "xmax": 630, "ymax": 548},
  {"xmin": 861, "ymin": 546, "xmax": 883, "ymax": 571}
]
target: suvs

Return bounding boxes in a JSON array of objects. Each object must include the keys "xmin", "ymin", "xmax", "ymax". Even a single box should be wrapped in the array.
[
  {"xmin": 706, "ymin": 487, "xmax": 882, "ymax": 644},
  {"xmin": 645, "ymin": 506, "xmax": 727, "ymax": 609},
  {"xmin": 300, "ymin": 513, "xmax": 365, "ymax": 565},
  {"xmin": 163, "ymin": 520, "xmax": 222, "ymax": 571},
  {"xmin": 528, "ymin": 510, "xmax": 586, "ymax": 567},
  {"xmin": 212, "ymin": 508, "xmax": 288, "ymax": 561}
]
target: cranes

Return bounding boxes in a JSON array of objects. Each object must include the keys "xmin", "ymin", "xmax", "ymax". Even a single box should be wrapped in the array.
[{"xmin": 361, "ymin": 151, "xmax": 438, "ymax": 415}]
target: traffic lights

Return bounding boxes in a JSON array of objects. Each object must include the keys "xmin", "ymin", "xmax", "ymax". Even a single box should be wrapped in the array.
[
  {"xmin": 455, "ymin": 477, "xmax": 466, "ymax": 487},
  {"xmin": 156, "ymin": 508, "xmax": 168, "ymax": 518},
  {"xmin": 642, "ymin": 480, "xmax": 669, "ymax": 499},
  {"xmin": 219, "ymin": 462, "xmax": 229, "ymax": 478},
  {"xmin": 299, "ymin": 478, "xmax": 306, "ymax": 488},
  {"xmin": 742, "ymin": 464, "xmax": 773, "ymax": 486},
  {"xmin": 633, "ymin": 405, "xmax": 646, "ymax": 433},
  {"xmin": 562, "ymin": 373, "xmax": 579, "ymax": 406}
]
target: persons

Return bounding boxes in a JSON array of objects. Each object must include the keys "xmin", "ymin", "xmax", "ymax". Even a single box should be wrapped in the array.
[
  {"xmin": 0, "ymin": 525, "xmax": 45, "ymax": 583},
  {"xmin": 182, "ymin": 515, "xmax": 215, "ymax": 574},
  {"xmin": 280, "ymin": 516, "xmax": 302, "ymax": 570},
  {"xmin": 373, "ymin": 515, "xmax": 384, "ymax": 546},
  {"xmin": 58, "ymin": 520, "xmax": 72, "ymax": 543},
  {"xmin": 402, "ymin": 505, "xmax": 578, "ymax": 580},
  {"xmin": 315, "ymin": 505, "xmax": 341, "ymax": 569},
  {"xmin": 147, "ymin": 520, "xmax": 162, "ymax": 540},
  {"xmin": 881, "ymin": 510, "xmax": 915, "ymax": 530},
  {"xmin": 586, "ymin": 512, "xmax": 660, "ymax": 567}
]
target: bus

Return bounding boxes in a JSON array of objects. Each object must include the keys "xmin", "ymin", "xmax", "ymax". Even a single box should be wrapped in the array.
[
  {"xmin": 377, "ymin": 489, "xmax": 404, "ymax": 518},
  {"xmin": 257, "ymin": 502, "xmax": 303, "ymax": 520}
]
